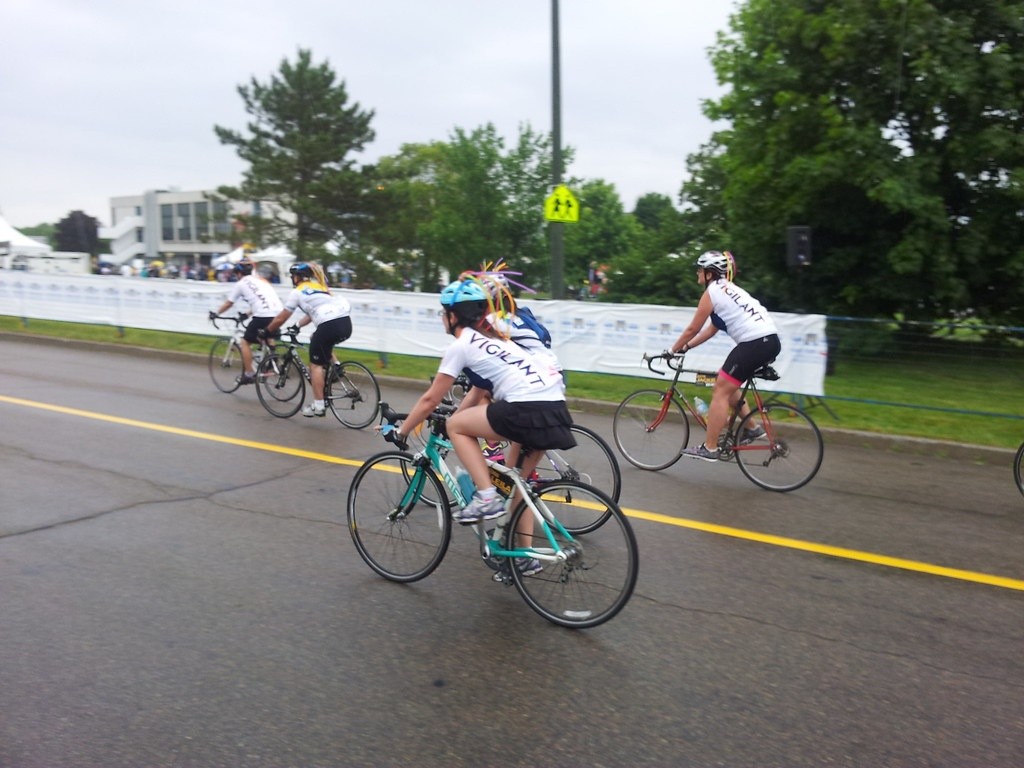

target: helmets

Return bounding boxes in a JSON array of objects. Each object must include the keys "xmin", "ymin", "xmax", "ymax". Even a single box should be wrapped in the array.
[
  {"xmin": 440, "ymin": 280, "xmax": 493, "ymax": 314},
  {"xmin": 697, "ymin": 251, "xmax": 728, "ymax": 273},
  {"xmin": 232, "ymin": 262, "xmax": 253, "ymax": 272},
  {"xmin": 478, "ymin": 272, "xmax": 512, "ymax": 296},
  {"xmin": 290, "ymin": 263, "xmax": 316, "ymax": 278}
]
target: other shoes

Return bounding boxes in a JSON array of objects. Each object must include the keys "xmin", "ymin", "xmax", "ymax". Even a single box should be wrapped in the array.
[
  {"xmin": 303, "ymin": 403, "xmax": 326, "ymax": 417},
  {"xmin": 265, "ymin": 354, "xmax": 279, "ymax": 369},
  {"xmin": 330, "ymin": 364, "xmax": 344, "ymax": 383},
  {"xmin": 240, "ymin": 372, "xmax": 256, "ymax": 384}
]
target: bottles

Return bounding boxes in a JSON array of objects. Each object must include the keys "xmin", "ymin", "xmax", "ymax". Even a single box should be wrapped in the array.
[
  {"xmin": 694, "ymin": 396, "xmax": 709, "ymax": 426},
  {"xmin": 456, "ymin": 465, "xmax": 477, "ymax": 504}
]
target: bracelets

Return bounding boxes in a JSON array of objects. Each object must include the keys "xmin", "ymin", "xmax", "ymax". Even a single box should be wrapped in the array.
[
  {"xmin": 686, "ymin": 344, "xmax": 691, "ymax": 349},
  {"xmin": 668, "ymin": 348, "xmax": 674, "ymax": 355}
]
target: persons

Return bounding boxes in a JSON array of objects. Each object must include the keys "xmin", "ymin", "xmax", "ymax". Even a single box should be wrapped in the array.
[
  {"xmin": 257, "ymin": 262, "xmax": 353, "ymax": 418},
  {"xmin": 663, "ymin": 250, "xmax": 781, "ymax": 463},
  {"xmin": 373, "ymin": 273, "xmax": 577, "ymax": 576},
  {"xmin": 340, "ymin": 272, "xmax": 349, "ymax": 283},
  {"xmin": 215, "ymin": 259, "xmax": 283, "ymax": 383},
  {"xmin": 94, "ymin": 260, "xmax": 237, "ymax": 282}
]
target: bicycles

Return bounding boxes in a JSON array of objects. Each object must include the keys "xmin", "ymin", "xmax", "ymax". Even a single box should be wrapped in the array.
[
  {"xmin": 611, "ymin": 348, "xmax": 825, "ymax": 493},
  {"xmin": 393, "ymin": 375, "xmax": 624, "ymax": 536},
  {"xmin": 253, "ymin": 328, "xmax": 381, "ymax": 430},
  {"xmin": 207, "ymin": 309, "xmax": 303, "ymax": 403},
  {"xmin": 346, "ymin": 401, "xmax": 641, "ymax": 631}
]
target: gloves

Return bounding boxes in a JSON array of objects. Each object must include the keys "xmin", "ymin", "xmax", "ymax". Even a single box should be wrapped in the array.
[
  {"xmin": 286, "ymin": 325, "xmax": 299, "ymax": 335},
  {"xmin": 238, "ymin": 313, "xmax": 247, "ymax": 320},
  {"xmin": 672, "ymin": 348, "xmax": 685, "ymax": 360},
  {"xmin": 208, "ymin": 313, "xmax": 217, "ymax": 319},
  {"xmin": 663, "ymin": 349, "xmax": 671, "ymax": 359},
  {"xmin": 257, "ymin": 327, "xmax": 270, "ymax": 339}
]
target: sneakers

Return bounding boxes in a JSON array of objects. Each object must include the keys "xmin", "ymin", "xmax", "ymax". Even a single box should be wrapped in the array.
[
  {"xmin": 680, "ymin": 442, "xmax": 721, "ymax": 462},
  {"xmin": 740, "ymin": 425, "xmax": 767, "ymax": 444},
  {"xmin": 479, "ymin": 442, "xmax": 506, "ymax": 461},
  {"xmin": 451, "ymin": 491, "xmax": 506, "ymax": 523},
  {"xmin": 492, "ymin": 553, "xmax": 542, "ymax": 582},
  {"xmin": 527, "ymin": 469, "xmax": 540, "ymax": 485}
]
